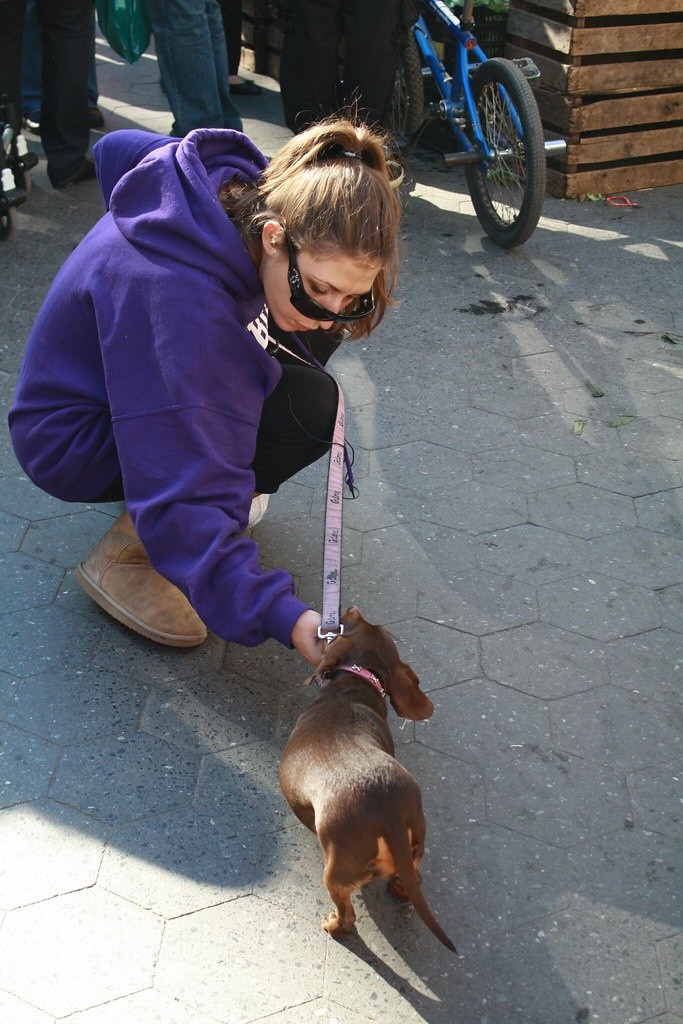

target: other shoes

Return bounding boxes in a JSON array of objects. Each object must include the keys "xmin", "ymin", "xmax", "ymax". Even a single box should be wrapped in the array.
[
  {"xmin": 26, "ymin": 111, "xmax": 43, "ymax": 136},
  {"xmin": 86, "ymin": 107, "xmax": 105, "ymax": 129},
  {"xmin": 228, "ymin": 74, "xmax": 262, "ymax": 94},
  {"xmin": 55, "ymin": 161, "xmax": 97, "ymax": 190}
]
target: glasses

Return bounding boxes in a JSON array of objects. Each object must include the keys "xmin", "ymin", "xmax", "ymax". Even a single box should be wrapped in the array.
[{"xmin": 281, "ymin": 223, "xmax": 378, "ymax": 325}]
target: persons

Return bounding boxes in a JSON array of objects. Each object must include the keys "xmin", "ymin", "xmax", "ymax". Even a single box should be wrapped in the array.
[
  {"xmin": 7, "ymin": 118, "xmax": 398, "ymax": 665},
  {"xmin": 216, "ymin": 0, "xmax": 262, "ymax": 96},
  {"xmin": 0, "ymin": 0, "xmax": 96, "ymax": 187},
  {"xmin": 142, "ymin": 0, "xmax": 244, "ymax": 137},
  {"xmin": 20, "ymin": 0, "xmax": 104, "ymax": 132},
  {"xmin": 275, "ymin": 0, "xmax": 417, "ymax": 134}
]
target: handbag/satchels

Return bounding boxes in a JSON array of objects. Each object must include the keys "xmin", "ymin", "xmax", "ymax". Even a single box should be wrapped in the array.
[{"xmin": 98, "ymin": 0, "xmax": 152, "ymax": 65}]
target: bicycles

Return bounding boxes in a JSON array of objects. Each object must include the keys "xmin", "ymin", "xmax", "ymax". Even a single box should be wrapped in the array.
[{"xmin": 390, "ymin": 0, "xmax": 568, "ymax": 248}]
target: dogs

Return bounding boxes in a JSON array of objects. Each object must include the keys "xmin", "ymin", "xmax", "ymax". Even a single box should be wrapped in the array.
[{"xmin": 279, "ymin": 606, "xmax": 458, "ymax": 956}]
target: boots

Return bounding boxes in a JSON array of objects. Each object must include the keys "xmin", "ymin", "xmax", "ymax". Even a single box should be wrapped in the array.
[{"xmin": 75, "ymin": 506, "xmax": 210, "ymax": 645}]
